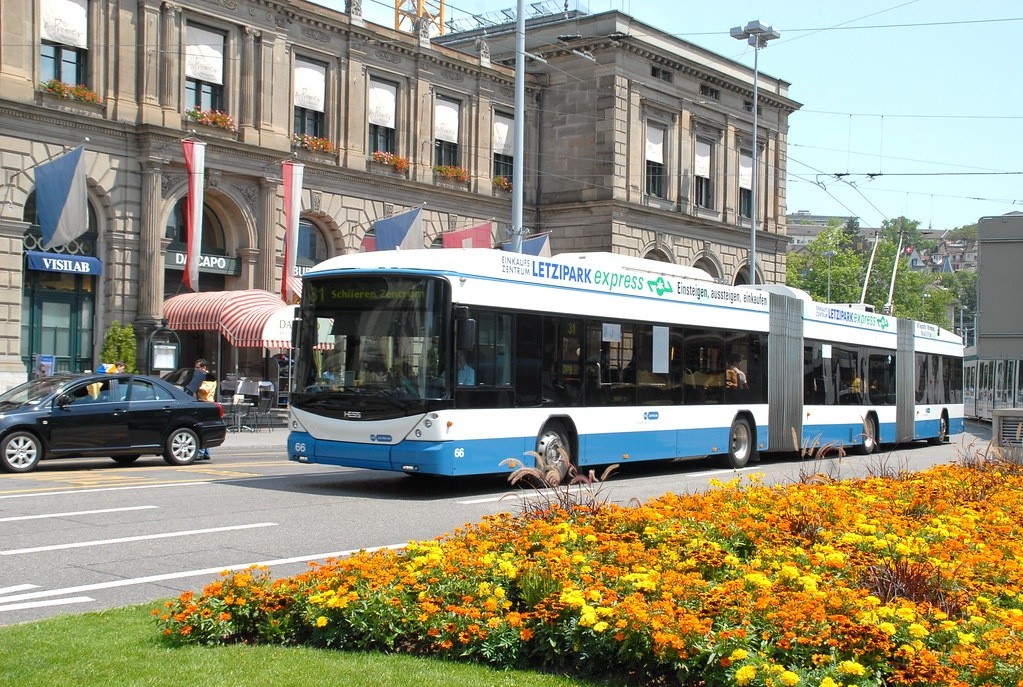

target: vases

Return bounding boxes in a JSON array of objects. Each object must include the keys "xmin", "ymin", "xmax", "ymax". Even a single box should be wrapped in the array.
[
  {"xmin": 34, "ymin": 90, "xmax": 106, "ymax": 120},
  {"xmin": 292, "ymin": 146, "xmax": 337, "ymax": 166},
  {"xmin": 492, "ymin": 188, "xmax": 527, "ymax": 203},
  {"xmin": 435, "ymin": 176, "xmax": 469, "ymax": 192},
  {"xmin": 184, "ymin": 120, "xmax": 240, "ymax": 141},
  {"xmin": 366, "ymin": 160, "xmax": 406, "ymax": 179}
]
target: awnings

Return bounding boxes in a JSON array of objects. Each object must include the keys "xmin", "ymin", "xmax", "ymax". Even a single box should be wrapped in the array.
[
  {"xmin": 162, "ymin": 278, "xmax": 337, "ymax": 350},
  {"xmin": 25, "ymin": 251, "xmax": 103, "ymax": 276}
]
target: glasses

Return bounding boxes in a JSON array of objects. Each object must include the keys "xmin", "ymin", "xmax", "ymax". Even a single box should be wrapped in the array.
[{"xmin": 41, "ymin": 371, "xmax": 45, "ymax": 373}]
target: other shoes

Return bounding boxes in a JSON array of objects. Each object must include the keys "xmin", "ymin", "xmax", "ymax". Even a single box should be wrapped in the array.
[
  {"xmin": 203, "ymin": 455, "xmax": 210, "ymax": 459},
  {"xmin": 195, "ymin": 456, "xmax": 201, "ymax": 461}
]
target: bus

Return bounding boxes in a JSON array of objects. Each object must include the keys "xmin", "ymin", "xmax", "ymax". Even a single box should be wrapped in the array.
[
  {"xmin": 288, "ymin": 247, "xmax": 966, "ymax": 488},
  {"xmin": 963, "ymin": 346, "xmax": 1023, "ymax": 422}
]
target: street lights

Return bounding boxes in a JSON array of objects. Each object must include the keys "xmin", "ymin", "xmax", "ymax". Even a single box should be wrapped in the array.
[{"xmin": 731, "ymin": 20, "xmax": 782, "ymax": 285}]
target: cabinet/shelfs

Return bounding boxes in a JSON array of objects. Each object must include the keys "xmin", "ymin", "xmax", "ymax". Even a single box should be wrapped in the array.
[{"xmin": 268, "ymin": 358, "xmax": 295, "ymax": 408}]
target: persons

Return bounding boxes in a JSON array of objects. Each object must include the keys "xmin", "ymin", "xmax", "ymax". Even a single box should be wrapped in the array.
[
  {"xmin": 100, "ymin": 362, "xmax": 126, "ymax": 396},
  {"xmin": 38, "ymin": 364, "xmax": 51, "ymax": 406},
  {"xmin": 195, "ymin": 359, "xmax": 218, "ymax": 460},
  {"xmin": 321, "ymin": 365, "xmax": 339, "ymax": 384},
  {"xmin": 727, "ymin": 353, "xmax": 749, "ymax": 389},
  {"xmin": 440, "ymin": 348, "xmax": 474, "ymax": 385}
]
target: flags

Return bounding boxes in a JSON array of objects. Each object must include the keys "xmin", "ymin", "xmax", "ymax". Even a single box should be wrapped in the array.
[
  {"xmin": 34, "ymin": 143, "xmax": 89, "ymax": 249},
  {"xmin": 442, "ymin": 222, "xmax": 492, "ymax": 248},
  {"xmin": 281, "ymin": 162, "xmax": 304, "ymax": 305},
  {"xmin": 373, "ymin": 207, "xmax": 424, "ymax": 251},
  {"xmin": 502, "ymin": 234, "xmax": 552, "ymax": 258},
  {"xmin": 182, "ymin": 139, "xmax": 207, "ymax": 293}
]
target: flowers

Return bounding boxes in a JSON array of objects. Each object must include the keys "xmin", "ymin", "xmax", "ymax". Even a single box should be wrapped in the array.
[
  {"xmin": 39, "ymin": 79, "xmax": 105, "ymax": 104},
  {"xmin": 292, "ymin": 133, "xmax": 334, "ymax": 153},
  {"xmin": 491, "ymin": 175, "xmax": 513, "ymax": 193},
  {"xmin": 433, "ymin": 164, "xmax": 468, "ymax": 183},
  {"xmin": 185, "ymin": 105, "xmax": 238, "ymax": 132},
  {"xmin": 368, "ymin": 150, "xmax": 409, "ymax": 172}
]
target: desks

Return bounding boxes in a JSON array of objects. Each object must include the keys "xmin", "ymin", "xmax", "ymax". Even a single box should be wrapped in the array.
[{"xmin": 221, "ymin": 380, "xmax": 275, "ymax": 396}]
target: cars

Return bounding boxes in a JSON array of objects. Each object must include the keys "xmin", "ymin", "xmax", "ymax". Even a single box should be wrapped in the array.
[{"xmin": 0, "ymin": 373, "xmax": 227, "ymax": 473}]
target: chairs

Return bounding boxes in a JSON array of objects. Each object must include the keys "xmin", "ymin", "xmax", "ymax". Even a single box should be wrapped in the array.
[
  {"xmin": 97, "ymin": 383, "xmax": 110, "ymax": 402},
  {"xmin": 676, "ymin": 368, "xmax": 719, "ymax": 404},
  {"xmin": 71, "ymin": 387, "xmax": 88, "ymax": 397},
  {"xmin": 221, "ymin": 398, "xmax": 274, "ymax": 433}
]
